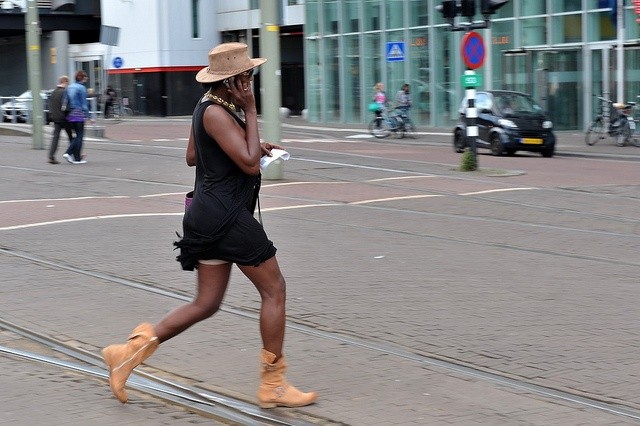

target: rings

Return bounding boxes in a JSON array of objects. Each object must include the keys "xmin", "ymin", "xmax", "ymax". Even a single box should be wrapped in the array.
[{"xmin": 244, "ymin": 88, "xmax": 247, "ymax": 90}]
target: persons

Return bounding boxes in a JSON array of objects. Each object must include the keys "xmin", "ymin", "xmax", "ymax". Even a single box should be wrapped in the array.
[
  {"xmin": 396, "ymin": 83, "xmax": 413, "ymax": 116},
  {"xmin": 46, "ymin": 74, "xmax": 73, "ymax": 163},
  {"xmin": 372, "ymin": 83, "xmax": 392, "ymax": 128},
  {"xmin": 104, "ymin": 85, "xmax": 118, "ymax": 117},
  {"xmin": 61, "ymin": 70, "xmax": 96, "ymax": 164},
  {"xmin": 101, "ymin": 40, "xmax": 318, "ymax": 408}
]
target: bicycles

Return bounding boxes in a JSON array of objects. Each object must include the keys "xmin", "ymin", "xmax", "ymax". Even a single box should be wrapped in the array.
[
  {"xmin": 370, "ymin": 105, "xmax": 405, "ymax": 138},
  {"xmin": 585, "ymin": 93, "xmax": 635, "ymax": 147}
]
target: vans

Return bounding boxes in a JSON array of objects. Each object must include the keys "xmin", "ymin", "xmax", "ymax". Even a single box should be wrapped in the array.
[{"xmin": 453, "ymin": 90, "xmax": 557, "ymax": 157}]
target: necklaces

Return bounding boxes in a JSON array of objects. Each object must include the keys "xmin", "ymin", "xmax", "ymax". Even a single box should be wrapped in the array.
[{"xmin": 204, "ymin": 92, "xmax": 236, "ymax": 111}]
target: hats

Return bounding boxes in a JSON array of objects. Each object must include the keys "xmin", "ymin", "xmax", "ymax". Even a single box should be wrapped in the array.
[{"xmin": 195, "ymin": 41, "xmax": 268, "ymax": 84}]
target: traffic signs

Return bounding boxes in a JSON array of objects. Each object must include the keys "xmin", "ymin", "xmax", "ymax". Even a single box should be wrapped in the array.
[
  {"xmin": 461, "ymin": 31, "xmax": 486, "ymax": 69},
  {"xmin": 384, "ymin": 41, "xmax": 410, "ymax": 64},
  {"xmin": 113, "ymin": 54, "xmax": 122, "ymax": 70}
]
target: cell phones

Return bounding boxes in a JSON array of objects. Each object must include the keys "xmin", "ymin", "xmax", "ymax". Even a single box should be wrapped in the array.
[{"xmin": 224, "ymin": 76, "xmax": 234, "ymax": 88}]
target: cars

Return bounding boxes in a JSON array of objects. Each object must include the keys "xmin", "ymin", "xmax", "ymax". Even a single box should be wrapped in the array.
[{"xmin": 1, "ymin": 89, "xmax": 53, "ymax": 125}]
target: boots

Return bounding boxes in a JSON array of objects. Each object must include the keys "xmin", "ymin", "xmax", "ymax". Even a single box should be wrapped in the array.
[
  {"xmin": 100, "ymin": 321, "xmax": 161, "ymax": 404},
  {"xmin": 255, "ymin": 348, "xmax": 318, "ymax": 410}
]
